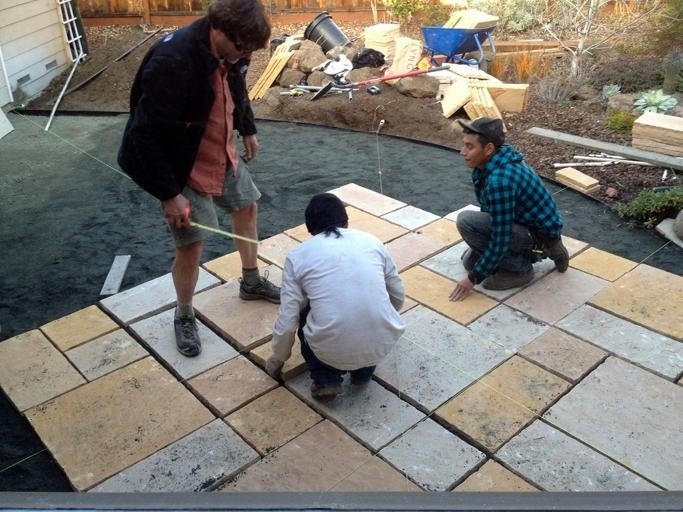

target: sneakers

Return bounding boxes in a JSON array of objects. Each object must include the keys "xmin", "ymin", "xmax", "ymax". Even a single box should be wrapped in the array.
[
  {"xmin": 238, "ymin": 276, "xmax": 280, "ymax": 304},
  {"xmin": 481, "ymin": 264, "xmax": 534, "ymax": 290},
  {"xmin": 174, "ymin": 308, "xmax": 201, "ymax": 357},
  {"xmin": 542, "ymin": 235, "xmax": 569, "ymax": 273},
  {"xmin": 310, "ymin": 382, "xmax": 344, "ymax": 397}
]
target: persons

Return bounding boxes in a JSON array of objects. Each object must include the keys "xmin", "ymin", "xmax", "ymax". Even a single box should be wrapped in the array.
[
  {"xmin": 266, "ymin": 192, "xmax": 405, "ymax": 399},
  {"xmin": 117, "ymin": 0, "xmax": 280, "ymax": 357},
  {"xmin": 447, "ymin": 117, "xmax": 569, "ymax": 301}
]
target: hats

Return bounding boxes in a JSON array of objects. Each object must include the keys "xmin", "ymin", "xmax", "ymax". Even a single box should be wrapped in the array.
[
  {"xmin": 305, "ymin": 194, "xmax": 347, "ymax": 231},
  {"xmin": 457, "ymin": 118, "xmax": 504, "ymax": 148}
]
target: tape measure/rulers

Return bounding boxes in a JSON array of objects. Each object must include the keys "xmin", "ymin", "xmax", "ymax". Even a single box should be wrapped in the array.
[{"xmin": 181, "ymin": 207, "xmax": 262, "ymax": 245}]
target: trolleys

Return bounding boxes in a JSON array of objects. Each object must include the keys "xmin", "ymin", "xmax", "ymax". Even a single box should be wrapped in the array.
[{"xmin": 418, "ymin": 22, "xmax": 498, "ymax": 74}]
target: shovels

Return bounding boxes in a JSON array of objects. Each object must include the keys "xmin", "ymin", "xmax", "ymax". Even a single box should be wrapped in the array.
[{"xmin": 311, "ymin": 65, "xmax": 451, "ymax": 100}]
